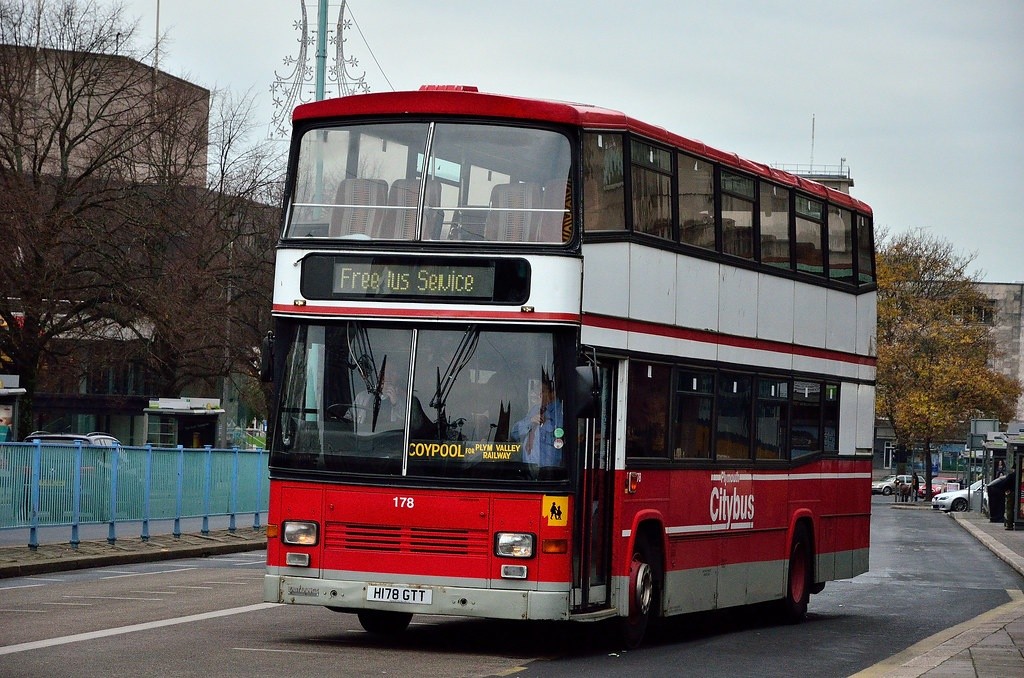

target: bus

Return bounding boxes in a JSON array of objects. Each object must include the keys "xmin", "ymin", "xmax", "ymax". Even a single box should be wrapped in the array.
[{"xmin": 259, "ymin": 83, "xmax": 877, "ymax": 646}]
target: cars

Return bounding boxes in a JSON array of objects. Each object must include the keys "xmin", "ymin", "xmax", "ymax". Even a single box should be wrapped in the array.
[
  {"xmin": 931, "ymin": 479, "xmax": 985, "ymax": 513},
  {"xmin": 871, "ymin": 474, "xmax": 926, "ymax": 496},
  {"xmin": 917, "ymin": 477, "xmax": 959, "ymax": 499}
]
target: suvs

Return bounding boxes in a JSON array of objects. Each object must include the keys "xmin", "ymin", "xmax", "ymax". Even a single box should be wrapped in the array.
[{"xmin": 11, "ymin": 431, "xmax": 146, "ymax": 522}]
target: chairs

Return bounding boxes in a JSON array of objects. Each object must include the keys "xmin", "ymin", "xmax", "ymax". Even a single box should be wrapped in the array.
[
  {"xmin": 762, "ymin": 235, "xmax": 776, "ymax": 265},
  {"xmin": 448, "ymin": 205, "xmax": 491, "ymax": 240},
  {"xmin": 776, "ymin": 239, "xmax": 789, "ymax": 267},
  {"xmin": 538, "ymin": 178, "xmax": 601, "ymax": 242},
  {"xmin": 684, "ymin": 212, "xmax": 716, "ymax": 250},
  {"xmin": 381, "ymin": 179, "xmax": 442, "ymax": 240},
  {"xmin": 329, "ymin": 179, "xmax": 388, "ymax": 238},
  {"xmin": 734, "ymin": 226, "xmax": 753, "ymax": 260},
  {"xmin": 723, "ymin": 218, "xmax": 736, "ymax": 256},
  {"xmin": 796, "ymin": 241, "xmax": 815, "ymax": 272},
  {"xmin": 484, "ymin": 182, "xmax": 542, "ymax": 240}
]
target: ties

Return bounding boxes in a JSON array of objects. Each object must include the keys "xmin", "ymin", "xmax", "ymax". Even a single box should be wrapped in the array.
[
  {"xmin": 526, "ymin": 407, "xmax": 545, "ymax": 453},
  {"xmin": 372, "ymin": 394, "xmax": 382, "ymax": 432}
]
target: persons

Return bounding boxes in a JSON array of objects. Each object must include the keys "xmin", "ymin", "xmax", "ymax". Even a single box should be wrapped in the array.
[
  {"xmin": 344, "ymin": 356, "xmax": 423, "ymax": 434},
  {"xmin": 995, "ymin": 459, "xmax": 1006, "ymax": 478},
  {"xmin": 910, "ymin": 473, "xmax": 919, "ymax": 501},
  {"xmin": 511, "ymin": 369, "xmax": 563, "ymax": 468},
  {"xmin": 900, "ymin": 484, "xmax": 909, "ymax": 502}
]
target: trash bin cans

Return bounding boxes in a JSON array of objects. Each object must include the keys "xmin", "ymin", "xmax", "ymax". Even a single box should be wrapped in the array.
[
  {"xmin": 987, "ymin": 475, "xmax": 1007, "ymax": 523},
  {"xmin": 972, "ymin": 490, "xmax": 983, "ymax": 512}
]
